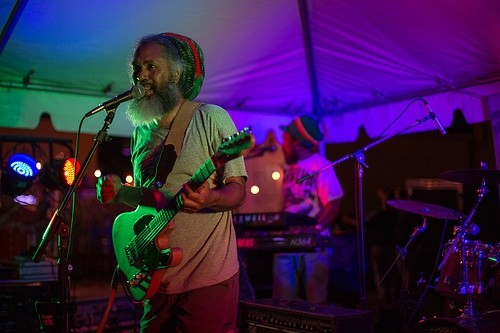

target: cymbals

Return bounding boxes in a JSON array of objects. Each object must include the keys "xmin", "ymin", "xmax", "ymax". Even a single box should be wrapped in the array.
[
  {"xmin": 440, "ymin": 169, "xmax": 500, "ymax": 184},
  {"xmin": 387, "ymin": 199, "xmax": 465, "ymax": 220}
]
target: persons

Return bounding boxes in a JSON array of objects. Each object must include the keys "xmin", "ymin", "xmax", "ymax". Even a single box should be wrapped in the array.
[
  {"xmin": 95, "ymin": 32, "xmax": 248, "ymax": 333},
  {"xmin": 341, "ymin": 188, "xmax": 411, "ymax": 302},
  {"xmin": 272, "ymin": 115, "xmax": 344, "ymax": 305}
]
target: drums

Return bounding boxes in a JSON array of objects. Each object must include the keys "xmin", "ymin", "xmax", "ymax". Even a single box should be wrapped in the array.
[
  {"xmin": 480, "ymin": 310, "xmax": 500, "ymax": 333},
  {"xmin": 436, "ymin": 239, "xmax": 497, "ymax": 297},
  {"xmin": 412, "ymin": 315, "xmax": 480, "ymax": 333}
]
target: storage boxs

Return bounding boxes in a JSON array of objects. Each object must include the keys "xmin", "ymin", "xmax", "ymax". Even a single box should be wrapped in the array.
[{"xmin": 0, "ymin": 259, "xmax": 59, "ymax": 282}]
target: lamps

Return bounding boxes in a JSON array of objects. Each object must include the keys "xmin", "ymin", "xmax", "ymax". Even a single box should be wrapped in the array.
[{"xmin": 4, "ymin": 154, "xmax": 81, "ymax": 196}]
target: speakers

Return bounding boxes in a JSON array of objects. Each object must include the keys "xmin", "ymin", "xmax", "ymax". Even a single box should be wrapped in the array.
[{"xmin": 236, "ymin": 298, "xmax": 372, "ymax": 333}]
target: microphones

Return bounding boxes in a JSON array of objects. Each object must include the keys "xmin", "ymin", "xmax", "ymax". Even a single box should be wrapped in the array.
[
  {"xmin": 451, "ymin": 209, "xmax": 480, "ymax": 236},
  {"xmin": 85, "ymin": 85, "xmax": 145, "ymax": 117},
  {"xmin": 422, "ymin": 100, "xmax": 446, "ymax": 136}
]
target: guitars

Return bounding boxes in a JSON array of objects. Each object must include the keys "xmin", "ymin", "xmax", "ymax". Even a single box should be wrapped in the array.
[{"xmin": 111, "ymin": 125, "xmax": 255, "ymax": 303}]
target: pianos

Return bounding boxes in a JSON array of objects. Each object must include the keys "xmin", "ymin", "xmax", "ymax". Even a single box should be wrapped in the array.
[{"xmin": 232, "ymin": 212, "xmax": 339, "ymax": 300}]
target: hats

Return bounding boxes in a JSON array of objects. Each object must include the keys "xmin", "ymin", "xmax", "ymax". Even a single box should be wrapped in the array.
[
  {"xmin": 280, "ymin": 115, "xmax": 323, "ymax": 147},
  {"xmin": 158, "ymin": 33, "xmax": 205, "ymax": 102}
]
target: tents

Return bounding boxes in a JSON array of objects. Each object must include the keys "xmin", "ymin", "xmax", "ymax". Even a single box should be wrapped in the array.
[{"xmin": 0, "ymin": 0, "xmax": 500, "ymax": 159}]
